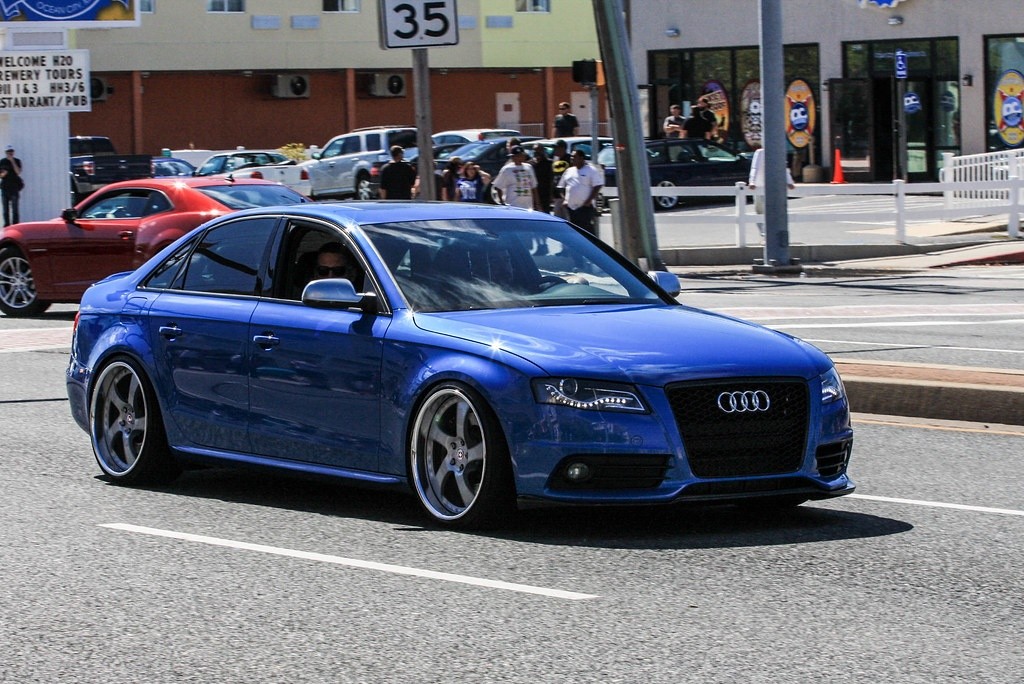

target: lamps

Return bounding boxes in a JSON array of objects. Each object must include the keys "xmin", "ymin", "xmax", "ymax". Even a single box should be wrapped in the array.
[
  {"xmin": 962, "ymin": 75, "xmax": 971, "ymax": 86},
  {"xmin": 664, "ymin": 28, "xmax": 680, "ymax": 36},
  {"xmin": 887, "ymin": 16, "xmax": 903, "ymax": 26}
]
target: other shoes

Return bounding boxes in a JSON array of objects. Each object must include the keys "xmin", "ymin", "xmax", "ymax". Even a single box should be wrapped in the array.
[{"xmin": 760, "ymin": 233, "xmax": 765, "ymax": 246}]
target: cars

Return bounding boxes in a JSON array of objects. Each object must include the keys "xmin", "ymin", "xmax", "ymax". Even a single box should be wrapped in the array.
[
  {"xmin": 0, "ymin": 174, "xmax": 317, "ymax": 320},
  {"xmin": 63, "ymin": 201, "xmax": 857, "ymax": 533},
  {"xmin": 432, "ymin": 133, "xmax": 619, "ymax": 202},
  {"xmin": 149, "ymin": 156, "xmax": 198, "ymax": 179},
  {"xmin": 193, "ymin": 150, "xmax": 313, "ymax": 197},
  {"xmin": 431, "ymin": 129, "xmax": 521, "ymax": 159}
]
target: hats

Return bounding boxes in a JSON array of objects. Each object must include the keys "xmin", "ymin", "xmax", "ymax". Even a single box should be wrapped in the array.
[
  {"xmin": 506, "ymin": 146, "xmax": 524, "ymax": 156},
  {"xmin": 3, "ymin": 143, "xmax": 15, "ymax": 151}
]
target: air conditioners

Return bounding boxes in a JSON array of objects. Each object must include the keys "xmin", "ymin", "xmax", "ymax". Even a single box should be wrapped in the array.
[
  {"xmin": 91, "ymin": 75, "xmax": 108, "ymax": 101},
  {"xmin": 271, "ymin": 75, "xmax": 311, "ymax": 98},
  {"xmin": 369, "ymin": 72, "xmax": 407, "ymax": 97}
]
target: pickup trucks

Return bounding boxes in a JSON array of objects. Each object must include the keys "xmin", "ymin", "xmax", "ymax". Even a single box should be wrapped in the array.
[{"xmin": 68, "ymin": 135, "xmax": 153, "ymax": 207}]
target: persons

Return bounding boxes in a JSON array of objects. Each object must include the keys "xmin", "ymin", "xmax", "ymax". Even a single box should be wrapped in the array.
[
  {"xmin": 432, "ymin": 233, "xmax": 588, "ymax": 305},
  {"xmin": 310, "ymin": 243, "xmax": 357, "ymax": 286},
  {"xmin": 0, "ymin": 144, "xmax": 23, "ymax": 227},
  {"xmin": 749, "ymin": 150, "xmax": 797, "ymax": 246},
  {"xmin": 106, "ymin": 192, "xmax": 150, "ymax": 217},
  {"xmin": 380, "ymin": 102, "xmax": 605, "ymax": 235},
  {"xmin": 664, "ymin": 99, "xmax": 721, "ymax": 142}
]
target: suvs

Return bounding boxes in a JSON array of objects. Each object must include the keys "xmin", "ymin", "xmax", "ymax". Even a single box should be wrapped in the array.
[
  {"xmin": 595, "ymin": 137, "xmax": 752, "ymax": 209},
  {"xmin": 296, "ymin": 125, "xmax": 439, "ymax": 202}
]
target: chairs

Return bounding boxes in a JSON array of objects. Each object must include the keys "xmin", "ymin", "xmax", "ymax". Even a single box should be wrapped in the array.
[
  {"xmin": 289, "ymin": 250, "xmax": 320, "ymax": 300},
  {"xmin": 432, "ymin": 244, "xmax": 484, "ymax": 305}
]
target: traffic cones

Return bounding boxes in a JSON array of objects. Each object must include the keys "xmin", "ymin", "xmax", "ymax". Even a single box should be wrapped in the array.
[{"xmin": 831, "ymin": 149, "xmax": 848, "ymax": 184}]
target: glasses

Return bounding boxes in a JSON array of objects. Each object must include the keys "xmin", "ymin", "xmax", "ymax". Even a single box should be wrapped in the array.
[
  {"xmin": 558, "ymin": 107, "xmax": 566, "ymax": 110},
  {"xmin": 317, "ymin": 266, "xmax": 349, "ymax": 277}
]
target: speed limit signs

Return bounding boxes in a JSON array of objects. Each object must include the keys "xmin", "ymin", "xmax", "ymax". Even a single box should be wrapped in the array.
[{"xmin": 377, "ymin": 0, "xmax": 460, "ymax": 51}]
